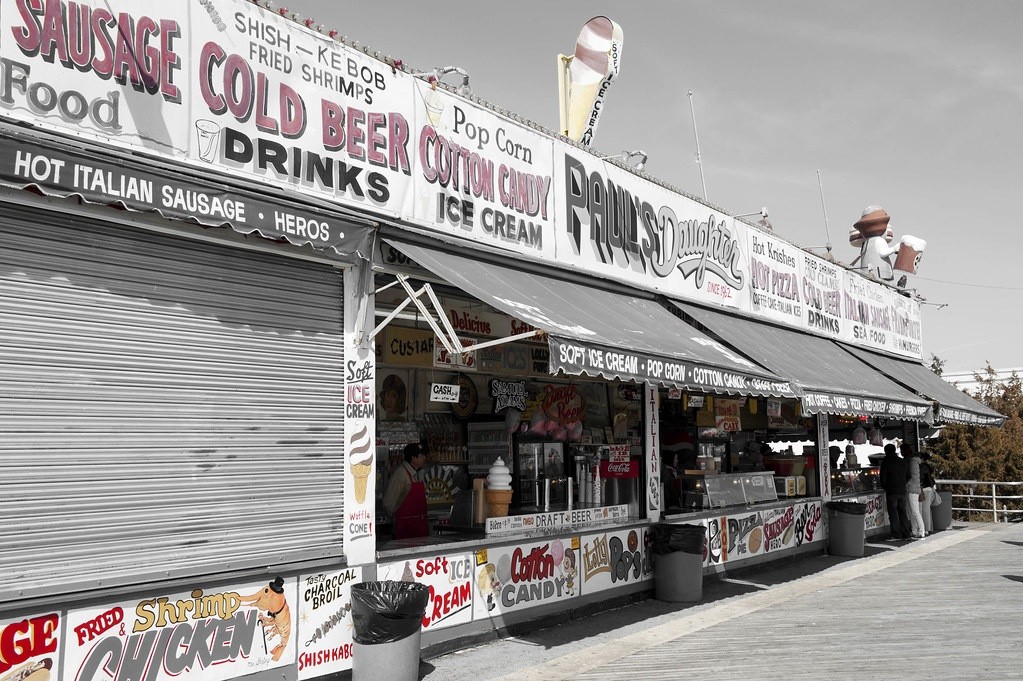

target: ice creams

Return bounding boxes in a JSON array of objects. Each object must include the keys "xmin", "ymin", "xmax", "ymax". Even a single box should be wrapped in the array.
[
  {"xmin": 486, "ymin": 457, "xmax": 513, "ymax": 517},
  {"xmin": 349, "ymin": 420, "xmax": 374, "ymax": 504}
]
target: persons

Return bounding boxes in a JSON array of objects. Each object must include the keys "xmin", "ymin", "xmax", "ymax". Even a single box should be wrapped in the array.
[
  {"xmin": 382, "ymin": 444, "xmax": 431, "ymax": 539},
  {"xmin": 660, "ymin": 453, "xmax": 685, "ymax": 508},
  {"xmin": 379, "ymin": 374, "xmax": 407, "ymax": 422},
  {"xmin": 828, "ymin": 446, "xmax": 844, "ymax": 468},
  {"xmin": 878, "ymin": 442, "xmax": 935, "ymax": 541}
]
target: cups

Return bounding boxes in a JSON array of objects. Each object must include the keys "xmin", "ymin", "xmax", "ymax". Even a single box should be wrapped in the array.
[{"xmin": 578, "ymin": 463, "xmax": 601, "ymax": 503}]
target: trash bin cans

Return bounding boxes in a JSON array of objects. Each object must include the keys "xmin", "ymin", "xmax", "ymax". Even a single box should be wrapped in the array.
[
  {"xmin": 649, "ymin": 524, "xmax": 707, "ymax": 602},
  {"xmin": 826, "ymin": 502, "xmax": 867, "ymax": 557},
  {"xmin": 349, "ymin": 581, "xmax": 429, "ymax": 681},
  {"xmin": 931, "ymin": 489, "xmax": 952, "ymax": 530}
]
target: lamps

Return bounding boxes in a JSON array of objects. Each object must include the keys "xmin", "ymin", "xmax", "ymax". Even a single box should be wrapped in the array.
[
  {"xmin": 731, "ymin": 206, "xmax": 773, "ymax": 231},
  {"xmin": 431, "ymin": 66, "xmax": 471, "ymax": 96},
  {"xmin": 798, "ymin": 243, "xmax": 835, "ymax": 261},
  {"xmin": 621, "ymin": 149, "xmax": 649, "ymax": 172},
  {"xmin": 894, "ymin": 288, "xmax": 917, "ymax": 299},
  {"xmin": 844, "ymin": 264, "xmax": 876, "ymax": 279}
]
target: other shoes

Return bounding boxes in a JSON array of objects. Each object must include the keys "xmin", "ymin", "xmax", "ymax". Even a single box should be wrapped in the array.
[
  {"xmin": 906, "ymin": 534, "xmax": 920, "ymax": 540},
  {"xmin": 886, "ymin": 536, "xmax": 903, "ymax": 541}
]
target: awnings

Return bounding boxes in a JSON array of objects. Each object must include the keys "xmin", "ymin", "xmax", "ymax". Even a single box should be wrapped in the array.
[{"xmin": 378, "ymin": 235, "xmax": 1008, "ymax": 431}]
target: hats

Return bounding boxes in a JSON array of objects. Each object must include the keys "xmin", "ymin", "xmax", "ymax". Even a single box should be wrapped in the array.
[
  {"xmin": 403, "ymin": 443, "xmax": 430, "ymax": 455},
  {"xmin": 829, "ymin": 446, "xmax": 845, "ymax": 453}
]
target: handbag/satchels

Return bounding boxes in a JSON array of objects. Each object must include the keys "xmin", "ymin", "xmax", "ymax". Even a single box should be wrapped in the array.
[
  {"xmin": 930, "ymin": 490, "xmax": 942, "ymax": 506},
  {"xmin": 918, "ymin": 489, "xmax": 925, "ymax": 502}
]
